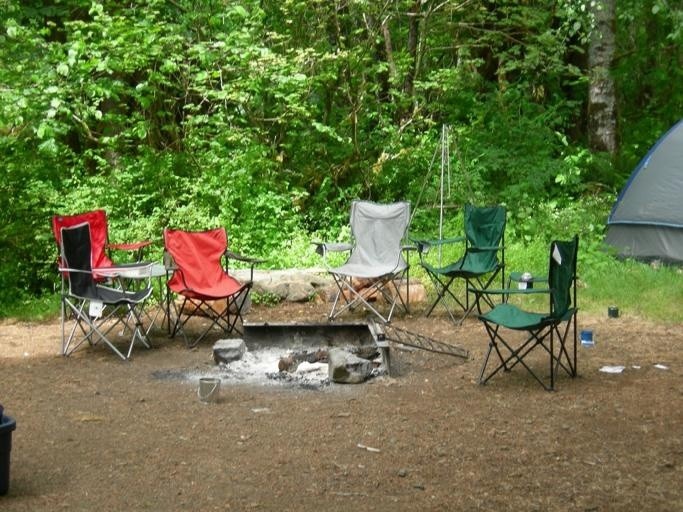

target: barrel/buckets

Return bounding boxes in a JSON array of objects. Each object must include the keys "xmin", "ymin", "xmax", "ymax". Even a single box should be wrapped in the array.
[
  {"xmin": 0, "ymin": 415, "xmax": 15, "ymax": 497},
  {"xmin": 198, "ymin": 378, "xmax": 221, "ymax": 402}
]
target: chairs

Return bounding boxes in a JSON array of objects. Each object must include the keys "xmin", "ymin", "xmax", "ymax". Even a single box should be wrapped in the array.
[
  {"xmin": 408, "ymin": 204, "xmax": 508, "ymax": 327},
  {"xmin": 51, "ymin": 209, "xmax": 268, "ymax": 361},
  {"xmin": 467, "ymin": 234, "xmax": 580, "ymax": 393},
  {"xmin": 311, "ymin": 201, "xmax": 417, "ymax": 325}
]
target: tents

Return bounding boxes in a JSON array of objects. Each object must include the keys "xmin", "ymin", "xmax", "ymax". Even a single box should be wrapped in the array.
[{"xmin": 596, "ymin": 119, "xmax": 683, "ymax": 266}]
